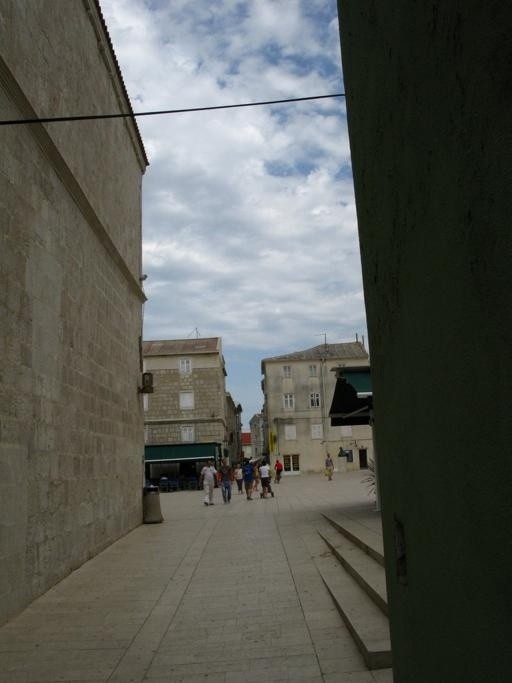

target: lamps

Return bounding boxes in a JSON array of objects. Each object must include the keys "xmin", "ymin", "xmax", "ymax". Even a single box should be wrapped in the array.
[
  {"xmin": 323, "ymin": 360, "xmax": 376, "ymax": 429},
  {"xmin": 137, "ymin": 373, "xmax": 153, "ymax": 393}
]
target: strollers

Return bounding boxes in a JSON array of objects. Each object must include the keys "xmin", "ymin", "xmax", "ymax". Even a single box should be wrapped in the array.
[{"xmin": 259, "ymin": 474, "xmax": 276, "ymax": 499}]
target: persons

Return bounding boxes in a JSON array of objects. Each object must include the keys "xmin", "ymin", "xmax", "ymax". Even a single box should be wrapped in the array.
[
  {"xmin": 325, "ymin": 453, "xmax": 334, "ymax": 480},
  {"xmin": 197, "ymin": 459, "xmax": 283, "ymax": 506}
]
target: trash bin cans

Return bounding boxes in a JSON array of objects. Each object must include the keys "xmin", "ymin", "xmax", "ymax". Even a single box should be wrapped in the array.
[{"xmin": 143, "ymin": 485, "xmax": 162, "ymax": 524}]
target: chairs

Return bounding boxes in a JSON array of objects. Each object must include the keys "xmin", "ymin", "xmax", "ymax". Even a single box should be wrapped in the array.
[{"xmin": 159, "ymin": 476, "xmax": 198, "ymax": 493}]
